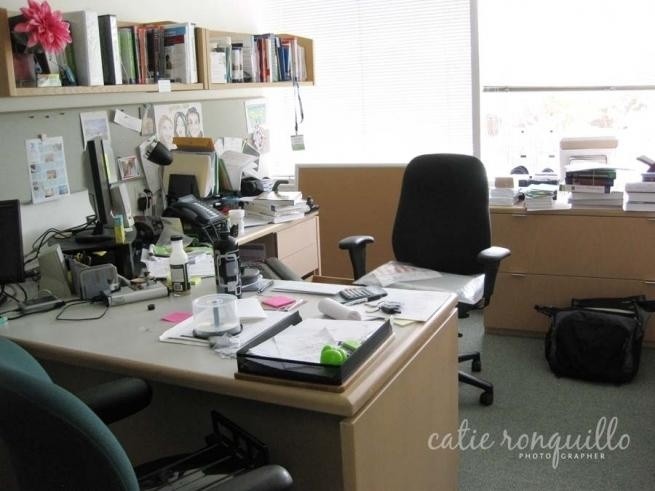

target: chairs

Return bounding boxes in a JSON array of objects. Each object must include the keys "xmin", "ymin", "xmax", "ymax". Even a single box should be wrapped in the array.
[
  {"xmin": 339, "ymin": 153, "xmax": 511, "ymax": 405},
  {"xmin": 0, "ymin": 336, "xmax": 293, "ymax": 491}
]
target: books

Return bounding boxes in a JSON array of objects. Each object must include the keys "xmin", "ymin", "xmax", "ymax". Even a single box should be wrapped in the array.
[
  {"xmin": 489, "ymin": 160, "xmax": 655, "ymax": 213},
  {"xmin": 245, "ymin": 190, "xmax": 310, "ymax": 224},
  {"xmin": 59, "ymin": 11, "xmax": 308, "ymax": 88}
]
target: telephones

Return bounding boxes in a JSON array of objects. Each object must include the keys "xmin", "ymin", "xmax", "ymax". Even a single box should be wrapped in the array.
[{"xmin": 170, "ymin": 193, "xmax": 226, "ymax": 229}]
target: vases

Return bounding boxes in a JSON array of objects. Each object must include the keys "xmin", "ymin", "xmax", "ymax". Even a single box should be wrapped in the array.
[{"xmin": 13, "ymin": 53, "xmax": 37, "ymax": 87}]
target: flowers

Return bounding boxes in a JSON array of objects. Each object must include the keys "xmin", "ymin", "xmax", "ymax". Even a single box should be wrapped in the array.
[{"xmin": 12, "ymin": 0, "xmax": 73, "ymax": 80}]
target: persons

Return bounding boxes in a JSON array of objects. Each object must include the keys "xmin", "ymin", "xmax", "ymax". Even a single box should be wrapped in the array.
[{"xmin": 159, "ymin": 107, "xmax": 203, "ymax": 150}]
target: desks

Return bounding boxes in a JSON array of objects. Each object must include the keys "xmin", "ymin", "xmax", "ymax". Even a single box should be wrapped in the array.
[
  {"xmin": 230, "ymin": 209, "xmax": 320, "ymax": 281},
  {"xmin": 484, "ymin": 190, "xmax": 655, "ymax": 348},
  {"xmin": 1, "ymin": 280, "xmax": 459, "ymax": 491}
]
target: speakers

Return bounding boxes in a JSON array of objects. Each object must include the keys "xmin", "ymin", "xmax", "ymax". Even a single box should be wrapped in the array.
[
  {"xmin": 110, "ymin": 182, "xmax": 135, "ymax": 228},
  {"xmin": 38, "ymin": 243, "xmax": 72, "ymax": 298}
]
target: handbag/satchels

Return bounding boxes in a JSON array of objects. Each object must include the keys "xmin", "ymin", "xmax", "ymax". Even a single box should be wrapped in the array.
[{"xmin": 534, "ymin": 296, "xmax": 655, "ymax": 386}]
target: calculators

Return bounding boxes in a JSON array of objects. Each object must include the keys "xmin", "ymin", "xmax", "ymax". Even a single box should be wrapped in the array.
[{"xmin": 339, "ymin": 286, "xmax": 386, "ymax": 301}]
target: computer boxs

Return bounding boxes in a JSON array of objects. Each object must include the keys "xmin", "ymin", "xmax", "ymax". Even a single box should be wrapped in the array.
[{"xmin": 48, "ymin": 225, "xmax": 146, "ymax": 285}]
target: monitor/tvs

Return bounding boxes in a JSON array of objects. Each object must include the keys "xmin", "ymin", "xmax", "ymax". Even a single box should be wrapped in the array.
[
  {"xmin": 0, "ymin": 199, "xmax": 25, "ymax": 304},
  {"xmin": 75, "ymin": 137, "xmax": 126, "ymax": 243}
]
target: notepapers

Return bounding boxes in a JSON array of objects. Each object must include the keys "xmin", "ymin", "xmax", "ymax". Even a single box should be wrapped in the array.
[{"xmin": 263, "ymin": 296, "xmax": 296, "ymax": 308}]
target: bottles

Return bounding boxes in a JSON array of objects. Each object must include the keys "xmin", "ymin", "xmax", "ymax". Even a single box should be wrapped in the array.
[
  {"xmin": 212, "ymin": 229, "xmax": 242, "ymax": 300},
  {"xmin": 168, "ymin": 234, "xmax": 191, "ymax": 293}
]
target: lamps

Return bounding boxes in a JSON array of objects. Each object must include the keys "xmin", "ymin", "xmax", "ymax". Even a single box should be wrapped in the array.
[{"xmin": 142, "ymin": 138, "xmax": 173, "ymax": 216}]
